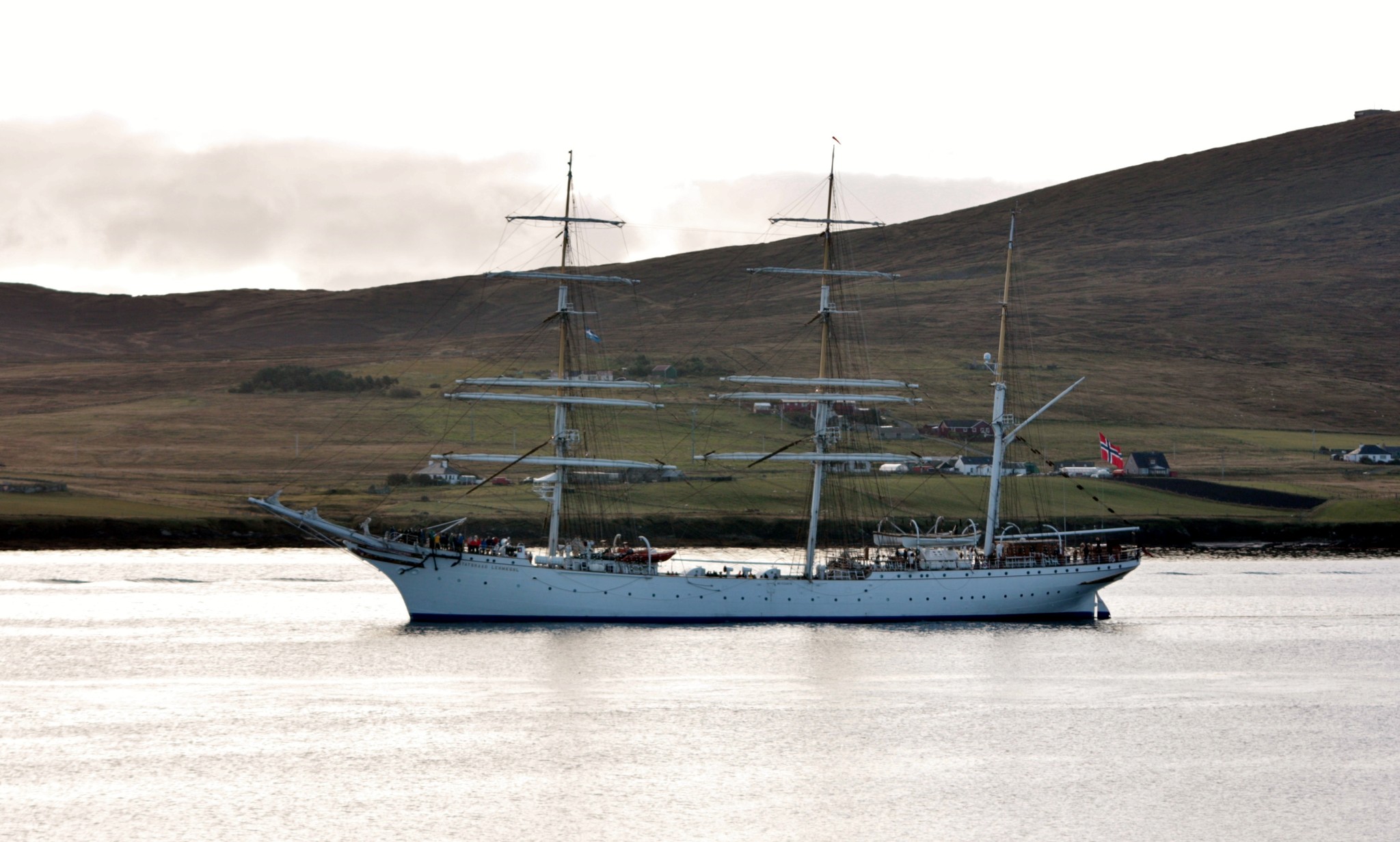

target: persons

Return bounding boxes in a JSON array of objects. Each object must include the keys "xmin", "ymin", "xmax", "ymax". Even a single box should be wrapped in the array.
[
  {"xmin": 980, "ymin": 542, "xmax": 1006, "ymax": 569},
  {"xmin": 705, "ymin": 570, "xmax": 779, "ymax": 578},
  {"xmin": 874, "ymin": 555, "xmax": 880, "ymax": 565},
  {"xmin": 1080, "ymin": 541, "xmax": 1122, "ymax": 563},
  {"xmin": 895, "ymin": 548, "xmax": 899, "ymax": 558},
  {"xmin": 388, "ymin": 524, "xmax": 533, "ymax": 566},
  {"xmin": 903, "ymin": 550, "xmax": 907, "ymax": 560}
]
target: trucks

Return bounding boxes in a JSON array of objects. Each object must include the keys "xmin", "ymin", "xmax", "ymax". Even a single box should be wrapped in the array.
[{"xmin": 493, "ymin": 477, "xmax": 511, "ymax": 486}]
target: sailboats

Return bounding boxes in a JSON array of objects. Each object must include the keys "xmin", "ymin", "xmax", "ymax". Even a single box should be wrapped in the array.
[{"xmin": 245, "ymin": 138, "xmax": 1141, "ymax": 626}]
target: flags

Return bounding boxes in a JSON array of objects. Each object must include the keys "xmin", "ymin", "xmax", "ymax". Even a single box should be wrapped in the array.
[
  {"xmin": 585, "ymin": 325, "xmax": 601, "ymax": 343},
  {"xmin": 1098, "ymin": 431, "xmax": 1123, "ymax": 470}
]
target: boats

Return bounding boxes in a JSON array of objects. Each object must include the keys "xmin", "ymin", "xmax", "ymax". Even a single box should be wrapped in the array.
[
  {"xmin": 570, "ymin": 546, "xmax": 676, "ymax": 564},
  {"xmin": 872, "ymin": 530, "xmax": 982, "ymax": 548}
]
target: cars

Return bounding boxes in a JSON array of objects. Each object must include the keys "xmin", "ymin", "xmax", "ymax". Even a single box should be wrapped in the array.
[{"xmin": 519, "ymin": 477, "xmax": 533, "ymax": 483}]
